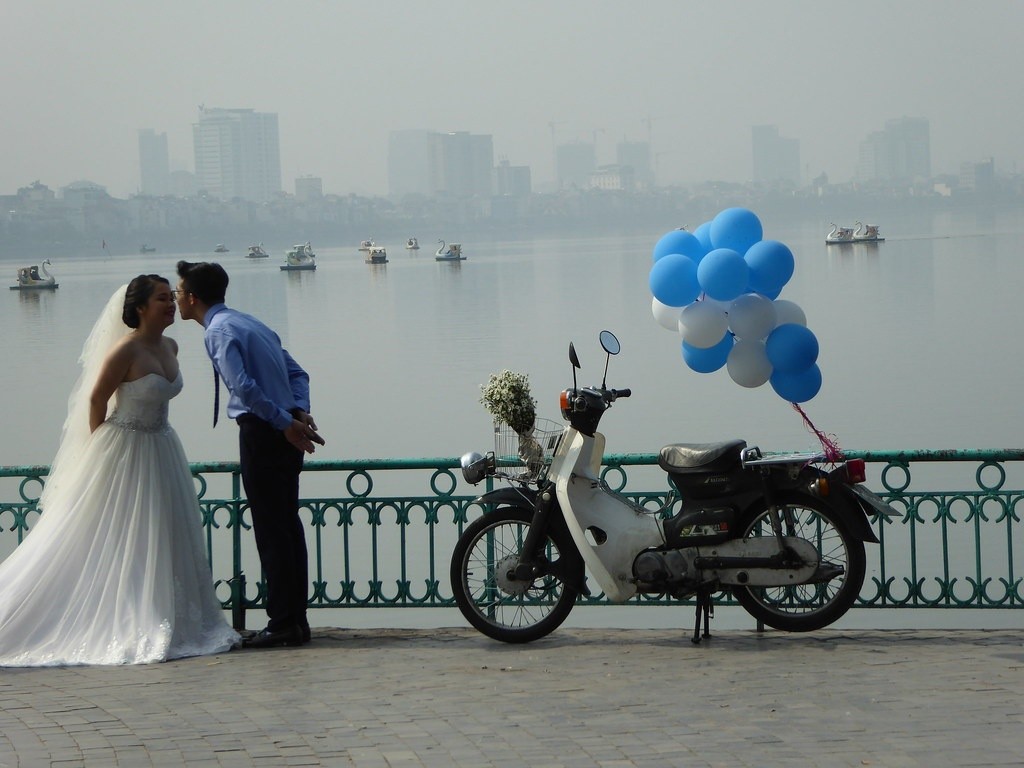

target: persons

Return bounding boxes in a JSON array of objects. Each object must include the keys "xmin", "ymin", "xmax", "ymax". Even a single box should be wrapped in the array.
[
  {"xmin": 174, "ymin": 260, "xmax": 325, "ymax": 647},
  {"xmin": 31, "ymin": 270, "xmax": 41, "ymax": 281},
  {"xmin": 373, "ymin": 250, "xmax": 382, "ymax": 253},
  {"xmin": 0, "ymin": 274, "xmax": 242, "ymax": 669}
]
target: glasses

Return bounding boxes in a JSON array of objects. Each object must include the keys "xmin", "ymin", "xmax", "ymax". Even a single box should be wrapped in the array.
[{"xmin": 170, "ymin": 290, "xmax": 198, "ymax": 301}]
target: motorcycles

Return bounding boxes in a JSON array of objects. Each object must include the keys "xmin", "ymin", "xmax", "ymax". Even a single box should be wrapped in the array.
[{"xmin": 449, "ymin": 329, "xmax": 905, "ymax": 644}]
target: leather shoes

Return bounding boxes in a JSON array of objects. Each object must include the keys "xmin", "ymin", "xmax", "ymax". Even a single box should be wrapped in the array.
[
  {"xmin": 303, "ymin": 625, "xmax": 312, "ymax": 642},
  {"xmin": 240, "ymin": 624, "xmax": 303, "ymax": 647}
]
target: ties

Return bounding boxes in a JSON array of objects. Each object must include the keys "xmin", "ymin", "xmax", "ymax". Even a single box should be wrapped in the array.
[{"xmin": 213, "ymin": 364, "xmax": 220, "ymax": 428}]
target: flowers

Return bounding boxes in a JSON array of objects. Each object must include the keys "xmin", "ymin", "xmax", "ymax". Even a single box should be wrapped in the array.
[{"xmin": 478, "ymin": 369, "xmax": 543, "ymax": 478}]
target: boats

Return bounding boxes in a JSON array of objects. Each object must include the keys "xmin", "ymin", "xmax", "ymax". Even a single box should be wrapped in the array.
[
  {"xmin": 214, "ymin": 243, "xmax": 230, "ymax": 253},
  {"xmin": 852, "ymin": 221, "xmax": 885, "ymax": 242},
  {"xmin": 279, "ymin": 241, "xmax": 318, "ymax": 270},
  {"xmin": 8, "ymin": 257, "xmax": 60, "ymax": 292},
  {"xmin": 356, "ymin": 236, "xmax": 376, "ymax": 252},
  {"xmin": 406, "ymin": 235, "xmax": 421, "ymax": 249},
  {"xmin": 368, "ymin": 246, "xmax": 387, "ymax": 263},
  {"xmin": 244, "ymin": 239, "xmax": 269, "ymax": 259},
  {"xmin": 433, "ymin": 238, "xmax": 467, "ymax": 261},
  {"xmin": 825, "ymin": 221, "xmax": 855, "ymax": 243}
]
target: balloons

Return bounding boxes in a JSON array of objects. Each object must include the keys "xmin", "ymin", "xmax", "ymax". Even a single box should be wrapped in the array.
[{"xmin": 649, "ymin": 207, "xmax": 822, "ymax": 404}]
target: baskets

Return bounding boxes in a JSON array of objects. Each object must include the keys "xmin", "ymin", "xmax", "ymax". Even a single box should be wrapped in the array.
[{"xmin": 494, "ymin": 418, "xmax": 566, "ymax": 485}]
